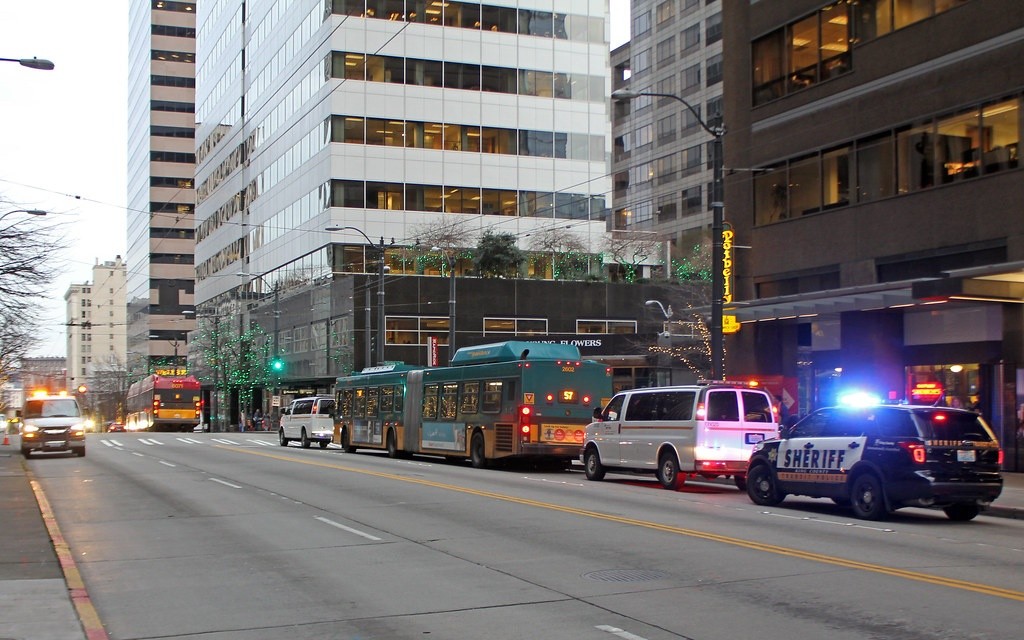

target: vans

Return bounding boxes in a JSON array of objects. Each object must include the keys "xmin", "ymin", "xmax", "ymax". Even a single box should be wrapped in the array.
[
  {"xmin": 578, "ymin": 378, "xmax": 779, "ymax": 491},
  {"xmin": 17, "ymin": 396, "xmax": 85, "ymax": 457},
  {"xmin": 277, "ymin": 397, "xmax": 335, "ymax": 448}
]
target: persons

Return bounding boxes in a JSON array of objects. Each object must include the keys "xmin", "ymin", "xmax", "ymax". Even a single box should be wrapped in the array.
[
  {"xmin": 345, "ymin": 388, "xmax": 476, "ymax": 419},
  {"xmin": 239, "ymin": 409, "xmax": 245, "ymax": 432},
  {"xmin": 487, "ymin": 386, "xmax": 499, "ymax": 410},
  {"xmin": 261, "ymin": 410, "xmax": 271, "ymax": 431},
  {"xmin": 253, "ymin": 409, "xmax": 260, "ymax": 431},
  {"xmin": 651, "ymin": 402, "xmax": 665, "ymax": 419},
  {"xmin": 950, "ymin": 397, "xmax": 963, "ymax": 408},
  {"xmin": 387, "ymin": 337, "xmax": 413, "ymax": 344},
  {"xmin": 585, "ymin": 328, "xmax": 604, "ymax": 333},
  {"xmin": 774, "ymin": 395, "xmax": 789, "ymax": 431}
]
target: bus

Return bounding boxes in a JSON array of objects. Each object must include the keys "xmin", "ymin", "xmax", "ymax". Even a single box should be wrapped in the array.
[
  {"xmin": 330, "ymin": 340, "xmax": 613, "ymax": 473},
  {"xmin": 125, "ymin": 374, "xmax": 200, "ymax": 432}
]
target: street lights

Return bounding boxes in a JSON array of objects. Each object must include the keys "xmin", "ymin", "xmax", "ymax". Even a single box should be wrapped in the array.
[
  {"xmin": 430, "ymin": 246, "xmax": 455, "ymax": 366},
  {"xmin": 236, "ymin": 271, "xmax": 279, "ymax": 431},
  {"xmin": 147, "ymin": 334, "xmax": 179, "ymax": 377},
  {"xmin": 643, "ymin": 300, "xmax": 673, "ymax": 386},
  {"xmin": 181, "ymin": 310, "xmax": 220, "ymax": 433},
  {"xmin": 126, "ymin": 351, "xmax": 150, "ymax": 377},
  {"xmin": 611, "ymin": 88, "xmax": 724, "ymax": 381},
  {"xmin": 110, "ymin": 363, "xmax": 132, "ymax": 390},
  {"xmin": 325, "ymin": 225, "xmax": 385, "ymax": 366}
]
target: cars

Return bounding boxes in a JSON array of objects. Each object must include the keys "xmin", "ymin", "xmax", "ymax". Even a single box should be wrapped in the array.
[
  {"xmin": 0, "ymin": 413, "xmax": 7, "ymax": 431},
  {"xmin": 746, "ymin": 383, "xmax": 1006, "ymax": 523},
  {"xmin": 108, "ymin": 422, "xmax": 127, "ymax": 433}
]
